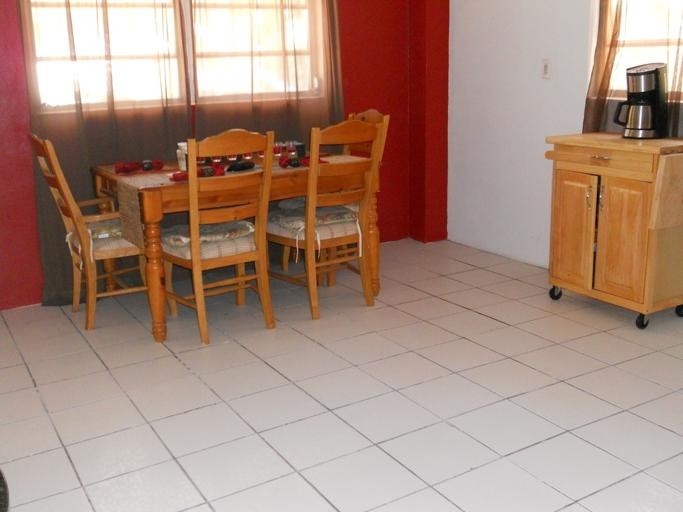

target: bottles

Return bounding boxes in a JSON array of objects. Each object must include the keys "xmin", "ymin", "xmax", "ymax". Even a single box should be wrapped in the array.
[{"xmin": 176, "ymin": 142, "xmax": 188, "ymax": 171}]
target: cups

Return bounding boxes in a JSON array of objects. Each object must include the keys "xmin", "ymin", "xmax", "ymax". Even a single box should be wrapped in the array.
[
  {"xmin": 274, "ymin": 141, "xmax": 282, "ymax": 157},
  {"xmin": 286, "ymin": 140, "xmax": 297, "ymax": 156}
]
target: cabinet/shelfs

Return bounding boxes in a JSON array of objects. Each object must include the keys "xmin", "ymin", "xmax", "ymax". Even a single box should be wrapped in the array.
[{"xmin": 543, "ymin": 132, "xmax": 682, "ymax": 315}]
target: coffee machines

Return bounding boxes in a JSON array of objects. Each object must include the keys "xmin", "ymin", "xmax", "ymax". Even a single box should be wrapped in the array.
[{"xmin": 613, "ymin": 63, "xmax": 667, "ymax": 140}]
